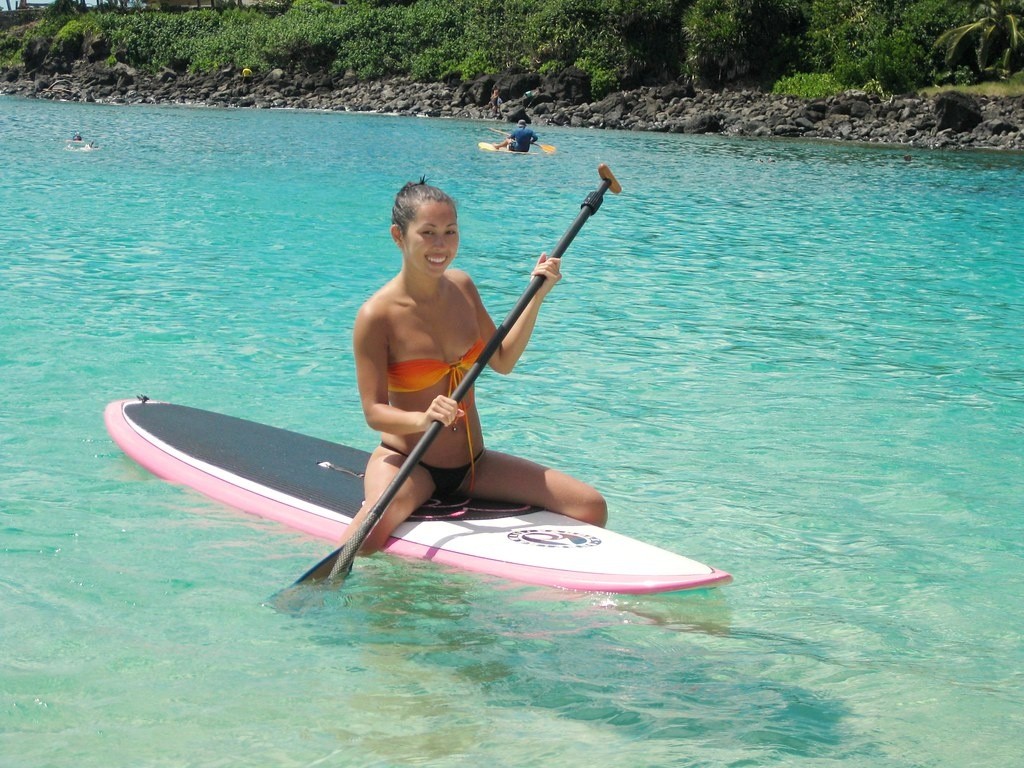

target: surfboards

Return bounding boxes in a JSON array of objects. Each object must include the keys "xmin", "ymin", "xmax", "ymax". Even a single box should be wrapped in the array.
[{"xmin": 101, "ymin": 396, "xmax": 733, "ymax": 596}]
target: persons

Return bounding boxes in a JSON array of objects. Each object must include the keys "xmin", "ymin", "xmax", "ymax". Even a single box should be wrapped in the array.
[
  {"xmin": 492, "ymin": 119, "xmax": 538, "ymax": 153},
  {"xmin": 242, "ymin": 68, "xmax": 253, "ymax": 83},
  {"xmin": 490, "ymin": 88, "xmax": 503, "ymax": 113},
  {"xmin": 344, "ymin": 174, "xmax": 608, "ymax": 570},
  {"xmin": 65, "ymin": 135, "xmax": 97, "ymax": 153}
]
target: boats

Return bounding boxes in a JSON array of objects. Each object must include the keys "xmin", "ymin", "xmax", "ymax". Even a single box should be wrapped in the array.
[
  {"xmin": 104, "ymin": 394, "xmax": 733, "ymax": 594},
  {"xmin": 477, "ymin": 141, "xmax": 540, "ymax": 155}
]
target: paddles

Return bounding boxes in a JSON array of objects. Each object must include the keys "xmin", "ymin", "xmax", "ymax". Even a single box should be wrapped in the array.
[
  {"xmin": 490, "ymin": 128, "xmax": 556, "ymax": 153},
  {"xmin": 262, "ymin": 162, "xmax": 621, "ymax": 620}
]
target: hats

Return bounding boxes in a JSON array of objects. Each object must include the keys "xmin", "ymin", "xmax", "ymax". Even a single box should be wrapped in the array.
[{"xmin": 517, "ymin": 120, "xmax": 527, "ymax": 126}]
